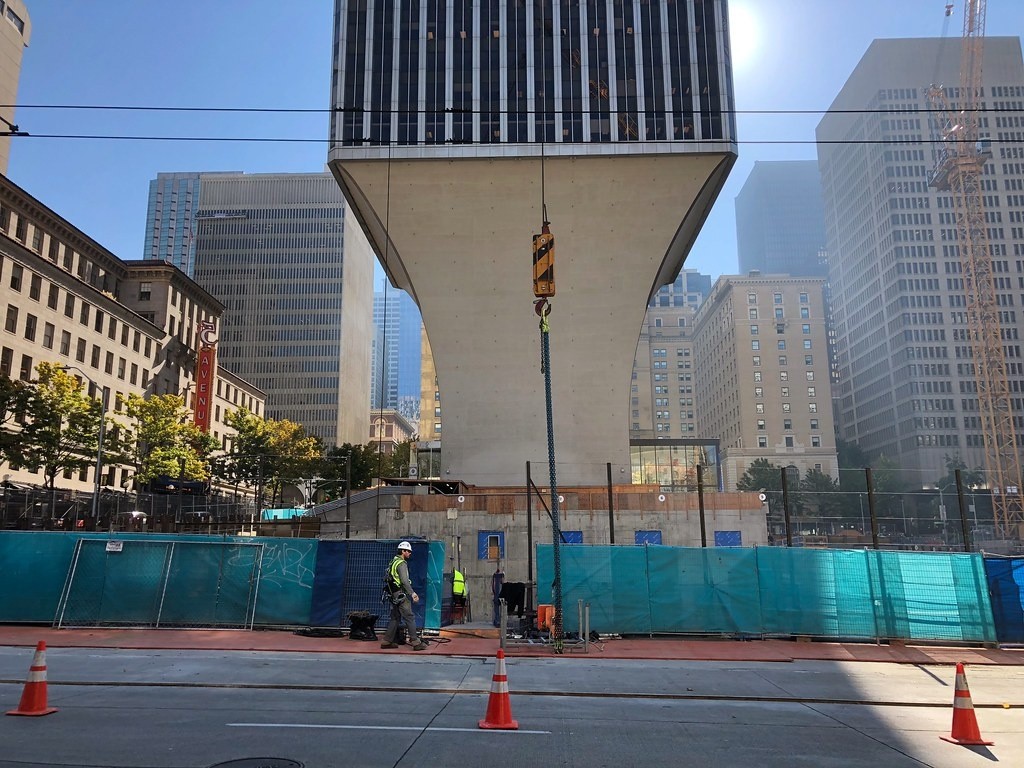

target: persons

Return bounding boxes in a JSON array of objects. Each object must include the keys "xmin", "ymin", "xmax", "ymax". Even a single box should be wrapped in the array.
[{"xmin": 381, "ymin": 541, "xmax": 429, "ymax": 651}]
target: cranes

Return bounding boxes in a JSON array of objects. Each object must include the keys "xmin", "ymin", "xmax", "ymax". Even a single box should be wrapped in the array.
[{"xmin": 923, "ymin": 0, "xmax": 1024, "ymax": 555}]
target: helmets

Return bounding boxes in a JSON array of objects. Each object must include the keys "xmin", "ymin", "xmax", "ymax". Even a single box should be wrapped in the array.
[{"xmin": 397, "ymin": 541, "xmax": 412, "ymax": 551}]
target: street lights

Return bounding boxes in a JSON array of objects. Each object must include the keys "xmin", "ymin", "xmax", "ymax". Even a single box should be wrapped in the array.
[{"xmin": 56, "ymin": 365, "xmax": 108, "ymax": 531}]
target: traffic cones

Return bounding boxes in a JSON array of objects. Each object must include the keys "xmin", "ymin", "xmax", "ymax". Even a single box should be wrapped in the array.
[
  {"xmin": 6, "ymin": 638, "xmax": 60, "ymax": 716},
  {"xmin": 938, "ymin": 661, "xmax": 996, "ymax": 745},
  {"xmin": 478, "ymin": 646, "xmax": 520, "ymax": 730}
]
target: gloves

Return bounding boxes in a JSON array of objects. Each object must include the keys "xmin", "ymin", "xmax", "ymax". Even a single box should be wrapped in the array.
[{"xmin": 412, "ymin": 592, "xmax": 419, "ymax": 603}]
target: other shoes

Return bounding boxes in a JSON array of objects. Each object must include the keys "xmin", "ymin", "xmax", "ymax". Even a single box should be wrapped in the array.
[
  {"xmin": 414, "ymin": 644, "xmax": 427, "ymax": 651},
  {"xmin": 381, "ymin": 643, "xmax": 398, "ymax": 649}
]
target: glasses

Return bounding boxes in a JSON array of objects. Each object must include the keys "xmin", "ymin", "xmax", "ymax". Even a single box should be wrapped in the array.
[{"xmin": 406, "ymin": 550, "xmax": 411, "ymax": 553}]
